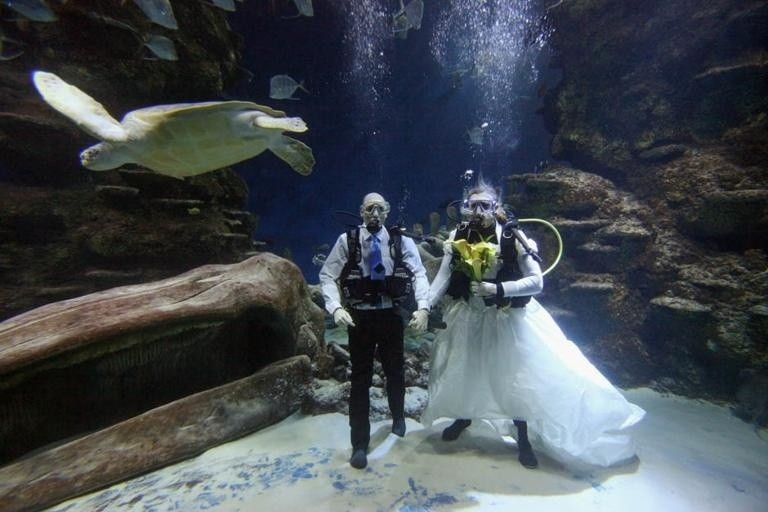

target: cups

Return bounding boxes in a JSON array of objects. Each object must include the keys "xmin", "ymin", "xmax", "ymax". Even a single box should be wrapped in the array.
[
  {"xmin": 464, "ymin": 197, "xmax": 499, "ymax": 213},
  {"xmin": 360, "ymin": 201, "xmax": 391, "ymax": 216}
]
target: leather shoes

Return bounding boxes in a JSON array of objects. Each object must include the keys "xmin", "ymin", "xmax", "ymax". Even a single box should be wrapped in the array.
[
  {"xmin": 333, "ymin": 309, "xmax": 356, "ymax": 328},
  {"xmin": 408, "ymin": 309, "xmax": 431, "ymax": 332},
  {"xmin": 471, "ymin": 280, "xmax": 498, "ymax": 298}
]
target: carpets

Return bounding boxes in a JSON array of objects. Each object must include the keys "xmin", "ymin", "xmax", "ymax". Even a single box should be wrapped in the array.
[{"xmin": 366, "ymin": 234, "xmax": 386, "ymax": 282}]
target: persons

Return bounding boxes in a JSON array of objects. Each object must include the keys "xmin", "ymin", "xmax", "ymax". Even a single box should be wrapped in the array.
[
  {"xmin": 408, "ymin": 186, "xmax": 544, "ymax": 468},
  {"xmin": 321, "ymin": 189, "xmax": 433, "ymax": 471}
]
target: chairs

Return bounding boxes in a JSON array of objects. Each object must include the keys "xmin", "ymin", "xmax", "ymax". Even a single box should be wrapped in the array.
[
  {"xmin": 518, "ymin": 440, "xmax": 537, "ymax": 469},
  {"xmin": 392, "ymin": 414, "xmax": 406, "ymax": 437},
  {"xmin": 443, "ymin": 419, "xmax": 472, "ymax": 441},
  {"xmin": 351, "ymin": 448, "xmax": 367, "ymax": 469}
]
